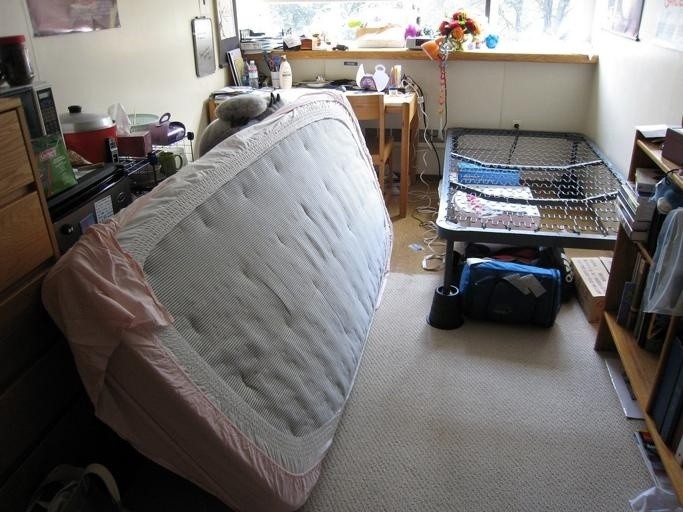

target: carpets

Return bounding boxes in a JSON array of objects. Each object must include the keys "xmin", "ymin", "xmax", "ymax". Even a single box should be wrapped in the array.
[{"xmin": 1, "ymin": 272, "xmax": 683, "ymax": 511}]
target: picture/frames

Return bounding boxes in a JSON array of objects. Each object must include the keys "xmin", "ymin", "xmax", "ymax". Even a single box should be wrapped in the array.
[{"xmin": 191, "ymin": 17, "xmax": 217, "ymax": 79}]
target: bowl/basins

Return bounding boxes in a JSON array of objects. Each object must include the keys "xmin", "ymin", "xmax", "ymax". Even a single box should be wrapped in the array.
[
  {"xmin": 136, "ymin": 163, "xmax": 162, "ymax": 182},
  {"xmin": 127, "ymin": 113, "xmax": 161, "ymax": 130}
]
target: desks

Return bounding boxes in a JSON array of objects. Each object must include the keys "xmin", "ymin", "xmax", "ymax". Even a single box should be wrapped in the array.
[{"xmin": 208, "ymin": 85, "xmax": 420, "ymax": 219}]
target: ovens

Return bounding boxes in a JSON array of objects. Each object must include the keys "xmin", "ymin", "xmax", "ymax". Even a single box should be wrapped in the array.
[{"xmin": 45, "ymin": 163, "xmax": 134, "ymax": 255}]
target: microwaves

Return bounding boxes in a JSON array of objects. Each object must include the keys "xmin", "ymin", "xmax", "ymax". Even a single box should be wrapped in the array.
[{"xmin": 0, "ymin": 79, "xmax": 67, "ymax": 147}]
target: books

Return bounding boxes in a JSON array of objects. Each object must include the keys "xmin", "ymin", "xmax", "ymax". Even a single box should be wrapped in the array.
[
  {"xmin": 613, "ymin": 166, "xmax": 667, "ymax": 242},
  {"xmin": 616, "ymin": 251, "xmax": 672, "ymax": 353}
]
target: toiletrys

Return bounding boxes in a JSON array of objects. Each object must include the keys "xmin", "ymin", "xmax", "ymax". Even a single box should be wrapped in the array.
[{"xmin": 278, "ymin": 56, "xmax": 292, "ymax": 88}]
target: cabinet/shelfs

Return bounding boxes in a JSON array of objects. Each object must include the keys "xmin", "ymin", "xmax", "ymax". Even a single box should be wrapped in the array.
[
  {"xmin": 595, "ymin": 134, "xmax": 683, "ymax": 512},
  {"xmin": 0, "ymin": 95, "xmax": 95, "ymax": 512}
]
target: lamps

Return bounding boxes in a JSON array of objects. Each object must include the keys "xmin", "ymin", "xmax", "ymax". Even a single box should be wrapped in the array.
[{"xmin": 421, "ymin": 35, "xmax": 445, "ymax": 62}]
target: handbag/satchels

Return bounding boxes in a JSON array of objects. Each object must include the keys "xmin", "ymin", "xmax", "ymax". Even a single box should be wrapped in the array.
[{"xmin": 458, "ymin": 258, "xmax": 562, "ymax": 328}]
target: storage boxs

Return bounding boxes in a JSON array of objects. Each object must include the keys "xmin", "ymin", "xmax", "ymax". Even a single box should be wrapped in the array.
[
  {"xmin": 571, "ymin": 255, "xmax": 612, "ymax": 325},
  {"xmin": 451, "ymin": 162, "xmax": 542, "ymax": 234}
]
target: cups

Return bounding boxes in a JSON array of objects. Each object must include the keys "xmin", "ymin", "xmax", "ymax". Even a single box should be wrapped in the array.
[{"xmin": 160, "ymin": 153, "xmax": 183, "ymax": 177}]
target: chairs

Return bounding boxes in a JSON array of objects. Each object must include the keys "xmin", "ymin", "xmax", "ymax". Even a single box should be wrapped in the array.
[{"xmin": 345, "ymin": 93, "xmax": 394, "ymax": 206}]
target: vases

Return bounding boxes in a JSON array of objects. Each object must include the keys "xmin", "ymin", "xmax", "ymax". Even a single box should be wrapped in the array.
[{"xmin": 447, "ymin": 38, "xmax": 467, "ymax": 51}]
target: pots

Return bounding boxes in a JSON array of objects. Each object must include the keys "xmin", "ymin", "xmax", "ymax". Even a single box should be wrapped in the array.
[{"xmin": 57, "ymin": 105, "xmax": 119, "ymax": 165}]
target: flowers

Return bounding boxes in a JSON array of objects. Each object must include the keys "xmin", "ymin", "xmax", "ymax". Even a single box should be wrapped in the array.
[{"xmin": 438, "ymin": 9, "xmax": 481, "ymax": 41}]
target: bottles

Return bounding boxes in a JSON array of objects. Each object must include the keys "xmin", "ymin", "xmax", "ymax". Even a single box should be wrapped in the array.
[{"xmin": 241, "ymin": 59, "xmax": 259, "ymax": 90}]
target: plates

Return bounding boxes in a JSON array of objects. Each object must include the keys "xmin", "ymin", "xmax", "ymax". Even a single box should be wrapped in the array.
[{"xmin": 303, "ymin": 81, "xmax": 329, "ymax": 88}]
